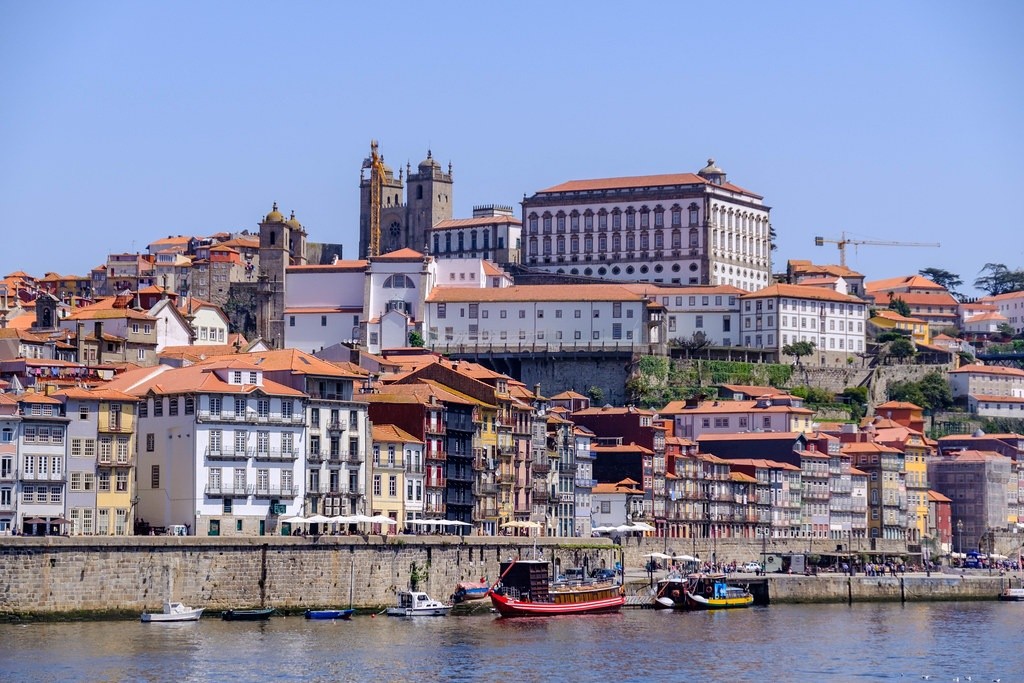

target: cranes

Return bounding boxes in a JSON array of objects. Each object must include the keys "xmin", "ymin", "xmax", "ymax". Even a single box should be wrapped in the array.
[{"xmin": 815, "ymin": 231, "xmax": 941, "ymax": 267}]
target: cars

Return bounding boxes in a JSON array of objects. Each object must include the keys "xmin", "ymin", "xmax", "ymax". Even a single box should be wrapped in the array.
[{"xmin": 742, "ymin": 562, "xmax": 763, "ymax": 574}]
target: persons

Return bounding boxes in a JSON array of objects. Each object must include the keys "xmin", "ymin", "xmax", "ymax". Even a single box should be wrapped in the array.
[
  {"xmin": 165, "ymin": 527, "xmax": 185, "ymax": 536},
  {"xmin": 5, "ymin": 528, "xmax": 10, "ymax": 535},
  {"xmin": 149, "ymin": 527, "xmax": 156, "ymax": 536},
  {"xmin": 613, "ymin": 555, "xmax": 1024, "ymax": 578},
  {"xmin": 11, "ymin": 527, "xmax": 22, "ymax": 535},
  {"xmin": 53, "ymin": 525, "xmax": 58, "ymax": 535},
  {"xmin": 288, "ymin": 526, "xmax": 643, "ymax": 547}
]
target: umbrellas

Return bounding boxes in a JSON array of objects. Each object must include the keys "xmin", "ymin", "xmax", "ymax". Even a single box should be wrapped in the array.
[
  {"xmin": 593, "ymin": 524, "xmax": 656, "ymax": 532},
  {"xmin": 642, "ymin": 551, "xmax": 701, "ymax": 562},
  {"xmin": 280, "ymin": 515, "xmax": 474, "ymax": 524},
  {"xmin": 501, "ymin": 520, "xmax": 543, "ymax": 529},
  {"xmin": 952, "ymin": 551, "xmax": 1008, "ymax": 561}
]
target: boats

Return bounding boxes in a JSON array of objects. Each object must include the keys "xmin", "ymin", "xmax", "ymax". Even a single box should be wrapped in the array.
[
  {"xmin": 221, "ymin": 607, "xmax": 276, "ymax": 622},
  {"xmin": 651, "ymin": 572, "xmax": 755, "ymax": 611},
  {"xmin": 489, "ymin": 559, "xmax": 629, "ymax": 618},
  {"xmin": 1001, "ymin": 588, "xmax": 1024, "ymax": 602},
  {"xmin": 141, "ymin": 602, "xmax": 206, "ymax": 622},
  {"xmin": 462, "ymin": 586, "xmax": 490, "ymax": 598},
  {"xmin": 387, "ymin": 590, "xmax": 453, "ymax": 616},
  {"xmin": 304, "ymin": 609, "xmax": 356, "ymax": 619}
]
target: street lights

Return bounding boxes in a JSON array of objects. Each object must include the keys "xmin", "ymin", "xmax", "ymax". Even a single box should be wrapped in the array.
[{"xmin": 957, "ymin": 519, "xmax": 964, "ymax": 568}]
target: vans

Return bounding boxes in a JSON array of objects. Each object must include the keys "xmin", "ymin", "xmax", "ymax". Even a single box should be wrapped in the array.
[{"xmin": 164, "ymin": 524, "xmax": 188, "ymax": 536}]
